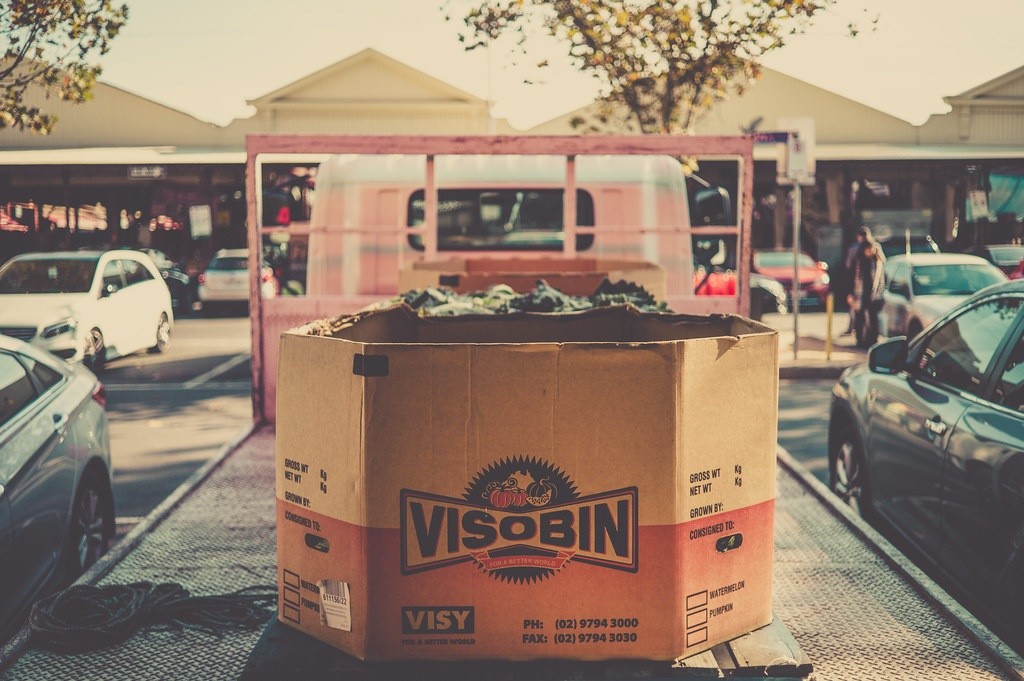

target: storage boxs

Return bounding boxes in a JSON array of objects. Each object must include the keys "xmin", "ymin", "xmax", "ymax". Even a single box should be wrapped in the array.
[{"xmin": 273, "ymin": 309, "xmax": 775, "ymax": 663}]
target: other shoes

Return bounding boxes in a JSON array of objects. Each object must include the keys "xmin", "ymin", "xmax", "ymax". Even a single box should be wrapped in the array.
[
  {"xmin": 850, "ymin": 343, "xmax": 871, "ymax": 350},
  {"xmin": 840, "ymin": 331, "xmax": 851, "ymax": 336}
]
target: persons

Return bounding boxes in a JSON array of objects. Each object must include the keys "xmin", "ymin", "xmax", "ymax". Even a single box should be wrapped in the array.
[
  {"xmin": 841, "ymin": 227, "xmax": 876, "ymax": 337},
  {"xmin": 845, "ymin": 241, "xmax": 885, "ymax": 350}
]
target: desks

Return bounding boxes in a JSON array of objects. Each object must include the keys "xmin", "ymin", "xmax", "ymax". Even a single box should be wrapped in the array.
[{"xmin": 239, "ymin": 607, "xmax": 818, "ymax": 681}]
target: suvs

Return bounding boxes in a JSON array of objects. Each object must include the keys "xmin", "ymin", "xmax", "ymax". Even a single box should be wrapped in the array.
[{"xmin": 199, "ymin": 247, "xmax": 279, "ymax": 313}]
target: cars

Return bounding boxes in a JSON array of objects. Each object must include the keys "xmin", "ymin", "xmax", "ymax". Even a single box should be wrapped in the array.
[
  {"xmin": 0, "ymin": 333, "xmax": 116, "ymax": 641},
  {"xmin": 872, "ymin": 251, "xmax": 1011, "ymax": 341},
  {"xmin": 973, "ymin": 244, "xmax": 1023, "ymax": 276},
  {"xmin": 750, "ymin": 273, "xmax": 788, "ymax": 324},
  {"xmin": 115, "ymin": 247, "xmax": 196, "ymax": 315},
  {"xmin": 0, "ymin": 249, "xmax": 174, "ymax": 372},
  {"xmin": 828, "ymin": 276, "xmax": 1024, "ymax": 659},
  {"xmin": 752, "ymin": 248, "xmax": 830, "ymax": 312}
]
